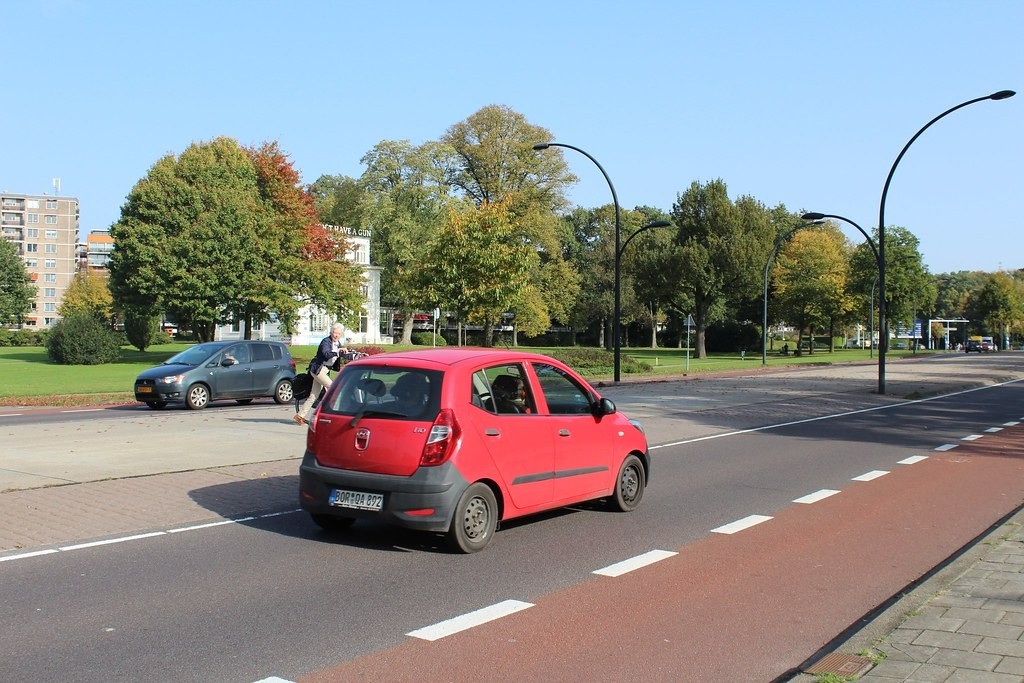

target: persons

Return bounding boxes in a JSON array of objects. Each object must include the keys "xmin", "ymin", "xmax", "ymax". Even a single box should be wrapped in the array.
[
  {"xmin": 294, "ymin": 323, "xmax": 370, "ymax": 426},
  {"xmin": 511, "ymin": 377, "xmax": 530, "ymax": 414},
  {"xmin": 390, "ymin": 372, "xmax": 429, "ymax": 415},
  {"xmin": 224, "ymin": 348, "xmax": 240, "ymax": 365},
  {"xmin": 780, "ymin": 342, "xmax": 788, "ymax": 356},
  {"xmin": 957, "ymin": 343, "xmax": 961, "ymax": 350}
]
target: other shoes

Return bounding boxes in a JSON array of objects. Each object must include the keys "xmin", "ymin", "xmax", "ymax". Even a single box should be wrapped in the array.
[{"xmin": 294, "ymin": 414, "xmax": 304, "ymax": 425}]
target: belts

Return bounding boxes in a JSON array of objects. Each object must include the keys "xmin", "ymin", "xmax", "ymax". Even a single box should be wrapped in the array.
[{"xmin": 324, "ymin": 365, "xmax": 331, "ymax": 369}]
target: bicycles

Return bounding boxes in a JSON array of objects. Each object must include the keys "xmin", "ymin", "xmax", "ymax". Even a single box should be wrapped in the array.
[{"xmin": 294, "ymin": 349, "xmax": 369, "ymax": 408}]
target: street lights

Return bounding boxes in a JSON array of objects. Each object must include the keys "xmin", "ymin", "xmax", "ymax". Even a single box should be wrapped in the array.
[
  {"xmin": 913, "ymin": 277, "xmax": 949, "ymax": 356},
  {"xmin": 532, "ymin": 143, "xmax": 672, "ymax": 387},
  {"xmin": 870, "ymin": 255, "xmax": 916, "ymax": 358},
  {"xmin": 763, "ymin": 220, "xmax": 826, "ymax": 365},
  {"xmin": 800, "ymin": 89, "xmax": 1018, "ymax": 393}
]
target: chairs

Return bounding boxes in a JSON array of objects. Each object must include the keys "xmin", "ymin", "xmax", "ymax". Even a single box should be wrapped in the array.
[
  {"xmin": 486, "ymin": 375, "xmax": 526, "ymax": 414},
  {"xmin": 358, "ymin": 379, "xmax": 386, "ymax": 409}
]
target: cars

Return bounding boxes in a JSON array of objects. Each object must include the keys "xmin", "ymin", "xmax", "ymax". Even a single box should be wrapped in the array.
[
  {"xmin": 965, "ymin": 336, "xmax": 998, "ymax": 354},
  {"xmin": 913, "ymin": 343, "xmax": 927, "ymax": 351},
  {"xmin": 298, "ymin": 347, "xmax": 651, "ymax": 554},
  {"xmin": 134, "ymin": 340, "xmax": 297, "ymax": 411},
  {"xmin": 891, "ymin": 343, "xmax": 909, "ymax": 351}
]
